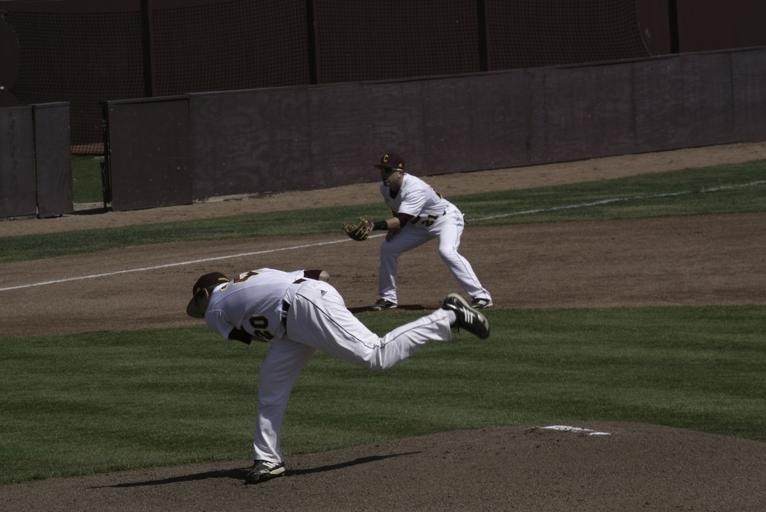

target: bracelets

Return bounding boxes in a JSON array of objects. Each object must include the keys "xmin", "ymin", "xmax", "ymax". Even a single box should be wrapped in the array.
[{"xmin": 373, "ymin": 220, "xmax": 388, "ymax": 231}]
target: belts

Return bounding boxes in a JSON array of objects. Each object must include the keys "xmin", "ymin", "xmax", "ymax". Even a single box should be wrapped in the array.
[{"xmin": 281, "ymin": 278, "xmax": 306, "ymax": 333}]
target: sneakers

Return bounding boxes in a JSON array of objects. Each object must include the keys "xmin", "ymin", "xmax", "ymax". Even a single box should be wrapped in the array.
[
  {"xmin": 367, "ymin": 297, "xmax": 394, "ymax": 310},
  {"xmin": 471, "ymin": 299, "xmax": 489, "ymax": 308},
  {"xmin": 245, "ymin": 461, "xmax": 286, "ymax": 482},
  {"xmin": 442, "ymin": 293, "xmax": 489, "ymax": 339}
]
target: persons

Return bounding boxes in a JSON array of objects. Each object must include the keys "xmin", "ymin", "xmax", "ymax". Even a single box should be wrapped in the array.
[
  {"xmin": 185, "ymin": 268, "xmax": 490, "ymax": 485},
  {"xmin": 362, "ymin": 150, "xmax": 494, "ymax": 314}
]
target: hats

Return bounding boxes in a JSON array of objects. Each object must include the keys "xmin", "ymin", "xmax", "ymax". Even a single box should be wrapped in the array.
[
  {"xmin": 375, "ymin": 151, "xmax": 407, "ymax": 172},
  {"xmin": 187, "ymin": 272, "xmax": 228, "ymax": 317}
]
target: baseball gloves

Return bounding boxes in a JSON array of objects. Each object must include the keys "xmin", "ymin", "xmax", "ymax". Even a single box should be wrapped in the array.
[{"xmin": 342, "ymin": 217, "xmax": 375, "ymax": 241}]
